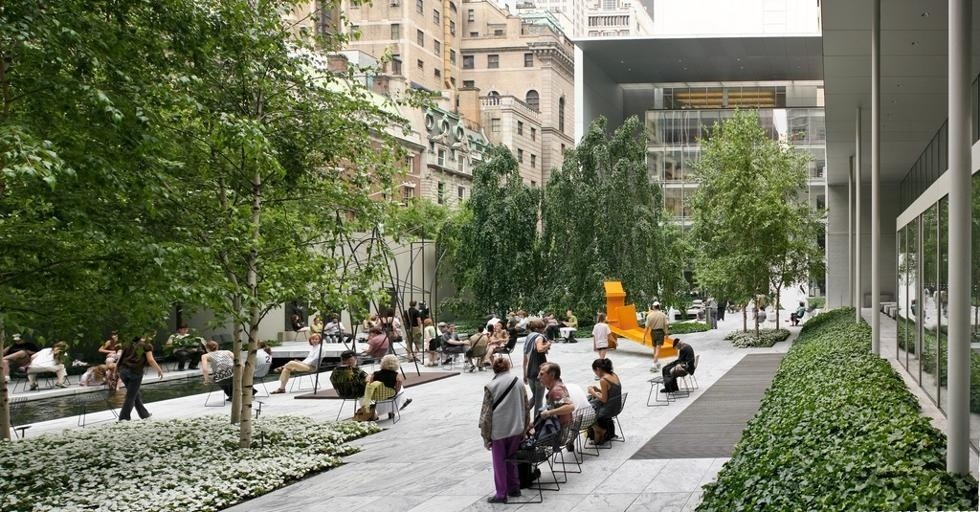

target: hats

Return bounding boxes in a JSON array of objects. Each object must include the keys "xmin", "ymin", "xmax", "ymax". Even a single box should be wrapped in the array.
[
  {"xmin": 11, "ymin": 333, "xmax": 23, "ymax": 341},
  {"xmin": 651, "ymin": 300, "xmax": 661, "ymax": 306},
  {"xmin": 672, "ymin": 338, "xmax": 681, "ymax": 349}
]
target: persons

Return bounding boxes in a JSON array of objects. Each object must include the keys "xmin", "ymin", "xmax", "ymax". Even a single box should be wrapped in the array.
[
  {"xmin": 522, "ymin": 319, "xmax": 550, "ymax": 427},
  {"xmin": 0, "ymin": 323, "xmax": 268, "ymax": 400},
  {"xmin": 330, "ymin": 351, "xmax": 378, "ymax": 421},
  {"xmin": 370, "ymin": 355, "xmax": 412, "ymax": 419},
  {"xmin": 752, "ymin": 292, "xmax": 769, "ymax": 323},
  {"xmin": 523, "ymin": 363, "xmax": 575, "ymax": 482},
  {"xmin": 659, "ymin": 338, "xmax": 694, "ymax": 392},
  {"xmin": 593, "ymin": 313, "xmax": 618, "ymax": 380},
  {"xmin": 584, "ymin": 356, "xmax": 622, "ymax": 444},
  {"xmin": 480, "ymin": 352, "xmax": 530, "ymax": 504},
  {"xmin": 270, "ymin": 332, "xmax": 325, "ymax": 395},
  {"xmin": 791, "ymin": 302, "xmax": 805, "ymax": 326},
  {"xmin": 299, "ymin": 299, "xmax": 580, "ymax": 372},
  {"xmin": 117, "ymin": 335, "xmax": 163, "ymax": 422},
  {"xmin": 641, "ymin": 302, "xmax": 667, "ymax": 371},
  {"xmin": 698, "ymin": 293, "xmax": 736, "ymax": 329}
]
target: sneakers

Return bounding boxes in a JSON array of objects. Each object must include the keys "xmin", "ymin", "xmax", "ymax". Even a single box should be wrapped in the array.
[
  {"xmin": 469, "ymin": 365, "xmax": 476, "ymax": 373},
  {"xmin": 29, "ymin": 382, "xmax": 38, "ymax": 391},
  {"xmin": 674, "ymin": 386, "xmax": 679, "ymax": 392},
  {"xmin": 487, "ymin": 495, "xmax": 507, "ymax": 503},
  {"xmin": 649, "ymin": 367, "xmax": 659, "ymax": 373},
  {"xmin": 507, "ymin": 487, "xmax": 522, "ymax": 497},
  {"xmin": 479, "ymin": 366, "xmax": 483, "ymax": 371},
  {"xmin": 54, "ymin": 382, "xmax": 69, "ymax": 389},
  {"xmin": 594, "ymin": 375, "xmax": 601, "ymax": 381},
  {"xmin": 656, "ymin": 363, "xmax": 662, "ymax": 370},
  {"xmin": 660, "ymin": 388, "xmax": 674, "ymax": 393}
]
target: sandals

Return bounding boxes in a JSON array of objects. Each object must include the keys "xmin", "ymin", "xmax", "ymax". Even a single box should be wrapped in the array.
[
  {"xmin": 273, "ymin": 366, "xmax": 284, "ymax": 374},
  {"xmin": 387, "ymin": 412, "xmax": 396, "ymax": 420},
  {"xmin": 269, "ymin": 388, "xmax": 285, "ymax": 395},
  {"xmin": 398, "ymin": 398, "xmax": 413, "ymax": 411}
]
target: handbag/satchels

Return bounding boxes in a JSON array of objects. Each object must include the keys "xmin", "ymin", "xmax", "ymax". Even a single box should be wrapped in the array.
[
  {"xmin": 429, "ymin": 335, "xmax": 443, "ymax": 351},
  {"xmin": 465, "ymin": 348, "xmax": 474, "ymax": 358},
  {"xmin": 410, "ymin": 326, "xmax": 423, "ymax": 346}
]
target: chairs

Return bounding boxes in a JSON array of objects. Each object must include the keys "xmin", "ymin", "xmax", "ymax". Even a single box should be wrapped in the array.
[
  {"xmin": 291, "ymin": 323, "xmax": 308, "ymax": 342},
  {"xmin": 147, "ymin": 348, "xmax": 194, "ymax": 374},
  {"xmin": 505, "ymin": 428, "xmax": 562, "ymax": 504},
  {"xmin": 554, "ymin": 405, "xmax": 599, "ymax": 463},
  {"xmin": 464, "ymin": 345, "xmax": 486, "ymax": 373},
  {"xmin": 9, "ymin": 398, "xmax": 32, "ymax": 440},
  {"xmin": 667, "ymin": 362, "xmax": 689, "ymax": 398},
  {"xmin": 290, "ymin": 351, "xmax": 326, "ymax": 393},
  {"xmin": 584, "ymin": 392, "xmax": 628, "ymax": 449},
  {"xmin": 78, "ymin": 376, "xmax": 120, "ymax": 428},
  {"xmin": 252, "ymin": 364, "xmax": 269, "ymax": 397},
  {"xmin": 205, "ymin": 363, "xmax": 239, "ymax": 407},
  {"xmin": 335, "ymin": 382, "xmax": 365, "ymax": 421},
  {"xmin": 439, "ymin": 335, "xmax": 464, "ymax": 370},
  {"xmin": 496, "ymin": 337, "xmax": 517, "ymax": 369},
  {"xmin": 676, "ymin": 355, "xmax": 700, "ymax": 393},
  {"xmin": 647, "ymin": 367, "xmax": 676, "ymax": 407},
  {"xmin": 529, "ymin": 414, "xmax": 582, "ymax": 485},
  {"xmin": 12, "ymin": 357, "xmax": 73, "ymax": 394},
  {"xmin": 369, "ymin": 380, "xmax": 405, "ymax": 424}
]
